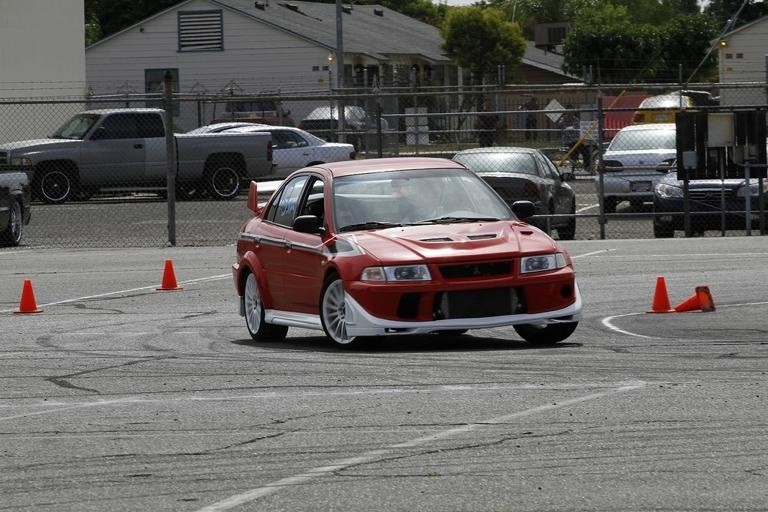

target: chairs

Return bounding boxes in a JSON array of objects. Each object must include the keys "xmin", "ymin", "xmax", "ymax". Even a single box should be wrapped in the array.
[
  {"xmin": 276, "ymin": 141, "xmax": 304, "ymax": 148},
  {"xmin": 308, "ymin": 196, "xmax": 364, "ymax": 224}
]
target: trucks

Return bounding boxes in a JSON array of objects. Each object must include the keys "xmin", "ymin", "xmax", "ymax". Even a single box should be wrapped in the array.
[{"xmin": 210, "ymin": 100, "xmax": 296, "ymax": 127}]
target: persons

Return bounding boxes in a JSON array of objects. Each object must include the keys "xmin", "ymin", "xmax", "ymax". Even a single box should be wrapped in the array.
[
  {"xmin": 517, "ymin": 96, "xmax": 541, "ymax": 142},
  {"xmin": 474, "ymin": 98, "xmax": 502, "ymax": 148},
  {"xmin": 400, "ymin": 175, "xmax": 449, "ymax": 225},
  {"xmin": 558, "ymin": 102, "xmax": 592, "ymax": 172}
]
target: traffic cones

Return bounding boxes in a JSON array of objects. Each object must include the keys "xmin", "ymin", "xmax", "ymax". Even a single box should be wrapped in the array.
[
  {"xmin": 645, "ymin": 276, "xmax": 677, "ymax": 313},
  {"xmin": 156, "ymin": 258, "xmax": 183, "ymax": 290},
  {"xmin": 675, "ymin": 285, "xmax": 716, "ymax": 313},
  {"xmin": 12, "ymin": 279, "xmax": 44, "ymax": 314}
]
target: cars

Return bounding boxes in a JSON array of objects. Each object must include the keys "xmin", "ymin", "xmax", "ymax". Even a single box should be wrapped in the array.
[
  {"xmin": 231, "ymin": 155, "xmax": 583, "ymax": 352},
  {"xmin": 652, "ymin": 156, "xmax": 768, "ymax": 238},
  {"xmin": 296, "ymin": 105, "xmax": 389, "ymax": 153},
  {"xmin": 595, "ymin": 122, "xmax": 677, "ymax": 213},
  {"xmin": 223, "ymin": 123, "xmax": 356, "ymax": 183},
  {"xmin": 631, "ymin": 94, "xmax": 698, "ymax": 125},
  {"xmin": 452, "ymin": 146, "xmax": 578, "ymax": 240}
]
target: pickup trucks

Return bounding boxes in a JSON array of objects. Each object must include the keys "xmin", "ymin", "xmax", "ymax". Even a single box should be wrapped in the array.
[{"xmin": 0, "ymin": 107, "xmax": 273, "ymax": 205}]
